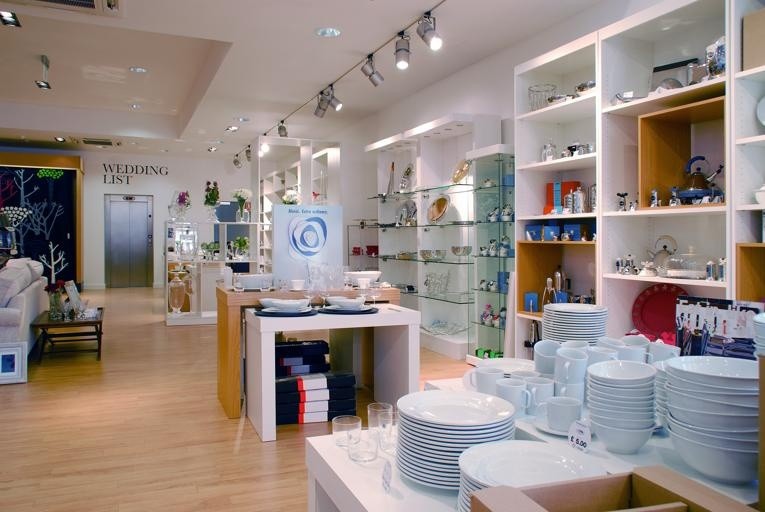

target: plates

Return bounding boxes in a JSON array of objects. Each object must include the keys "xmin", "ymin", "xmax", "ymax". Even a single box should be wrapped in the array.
[
  {"xmin": 394, "ymin": 389, "xmax": 611, "ymax": 512},
  {"xmin": 539, "ymin": 302, "xmax": 608, "ymax": 343},
  {"xmin": 749, "ymin": 308, "xmax": 765, "ymax": 353}
]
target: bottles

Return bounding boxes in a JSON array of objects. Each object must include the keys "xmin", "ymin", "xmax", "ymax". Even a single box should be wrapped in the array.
[{"xmin": 564, "ymin": 183, "xmax": 596, "ymax": 213}]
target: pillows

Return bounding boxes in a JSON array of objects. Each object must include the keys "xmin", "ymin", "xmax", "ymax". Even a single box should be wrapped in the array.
[{"xmin": 0, "ymin": 257, "xmax": 44, "ymax": 308}]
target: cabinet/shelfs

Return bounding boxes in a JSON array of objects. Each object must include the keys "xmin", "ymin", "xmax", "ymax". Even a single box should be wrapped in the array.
[
  {"xmin": 259, "ymin": 148, "xmax": 341, "ymax": 274},
  {"xmin": 305, "ymin": 378, "xmax": 759, "ymax": 512}
]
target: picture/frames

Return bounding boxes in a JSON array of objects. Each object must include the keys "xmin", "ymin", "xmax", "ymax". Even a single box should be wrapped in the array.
[
  {"xmin": 0, "ymin": 342, "xmax": 28, "ymax": 385},
  {"xmin": 64, "ymin": 280, "xmax": 82, "ymax": 312}
]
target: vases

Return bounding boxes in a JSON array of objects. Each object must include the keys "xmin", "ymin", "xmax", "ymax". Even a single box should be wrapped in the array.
[
  {"xmin": 235, "ymin": 210, "xmax": 249, "ymax": 223},
  {"xmin": 49, "ymin": 293, "xmax": 62, "ymax": 320},
  {"xmin": 207, "ymin": 206, "xmax": 220, "ymax": 223}
]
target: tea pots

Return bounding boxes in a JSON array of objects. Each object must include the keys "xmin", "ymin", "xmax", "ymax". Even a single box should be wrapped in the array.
[
  {"xmin": 646, "ymin": 234, "xmax": 677, "ymax": 272},
  {"xmin": 670, "ymin": 153, "xmax": 724, "ymax": 203}
]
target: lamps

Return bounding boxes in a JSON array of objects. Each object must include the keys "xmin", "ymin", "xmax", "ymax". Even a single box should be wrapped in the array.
[{"xmin": 234, "ymin": 0, "xmax": 447, "ymax": 169}]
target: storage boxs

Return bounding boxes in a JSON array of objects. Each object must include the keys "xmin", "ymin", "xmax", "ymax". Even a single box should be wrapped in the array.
[{"xmin": 470, "ymin": 465, "xmax": 761, "ymax": 512}]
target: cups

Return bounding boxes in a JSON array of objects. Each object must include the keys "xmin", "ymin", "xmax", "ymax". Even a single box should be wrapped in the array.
[
  {"xmin": 457, "ymin": 335, "xmax": 681, "ymax": 438},
  {"xmin": 528, "ymin": 82, "xmax": 557, "ymax": 111},
  {"xmin": 541, "ymin": 143, "xmax": 558, "ymax": 162},
  {"xmin": 232, "ymin": 274, "xmax": 370, "ymax": 292},
  {"xmin": 330, "ymin": 402, "xmax": 401, "ymax": 468}
]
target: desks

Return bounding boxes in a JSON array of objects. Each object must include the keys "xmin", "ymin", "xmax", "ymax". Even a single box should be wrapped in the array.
[
  {"xmin": 30, "ymin": 308, "xmax": 105, "ymax": 361},
  {"xmin": 216, "ymin": 287, "xmax": 400, "ymax": 419},
  {"xmin": 245, "ymin": 303, "xmax": 421, "ymax": 442}
]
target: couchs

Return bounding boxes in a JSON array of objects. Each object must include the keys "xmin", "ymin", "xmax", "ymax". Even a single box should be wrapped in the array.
[{"xmin": 0, "ymin": 276, "xmax": 48, "ymax": 355}]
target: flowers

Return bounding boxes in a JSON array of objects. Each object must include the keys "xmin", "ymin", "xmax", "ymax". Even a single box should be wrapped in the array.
[
  {"xmin": 202, "ymin": 182, "xmax": 219, "ymax": 205},
  {"xmin": 234, "ymin": 189, "xmax": 251, "ymax": 218},
  {"xmin": 45, "ymin": 281, "xmax": 65, "ymax": 293},
  {"xmin": 176, "ymin": 190, "xmax": 190, "ymax": 208}
]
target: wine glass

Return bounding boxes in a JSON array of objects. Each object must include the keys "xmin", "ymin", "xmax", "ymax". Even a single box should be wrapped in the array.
[{"xmin": 369, "ymin": 282, "xmax": 382, "ymax": 309}]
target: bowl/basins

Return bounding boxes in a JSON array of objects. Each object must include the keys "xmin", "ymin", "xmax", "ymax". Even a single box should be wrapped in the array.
[
  {"xmin": 347, "ymin": 271, "xmax": 382, "ymax": 285},
  {"xmin": 586, "ymin": 356, "xmax": 760, "ymax": 483},
  {"xmin": 547, "ymin": 81, "xmax": 595, "ymax": 108},
  {"xmin": 419, "ymin": 246, "xmax": 473, "ymax": 261},
  {"xmin": 258, "ymin": 296, "xmax": 370, "ymax": 312}
]
